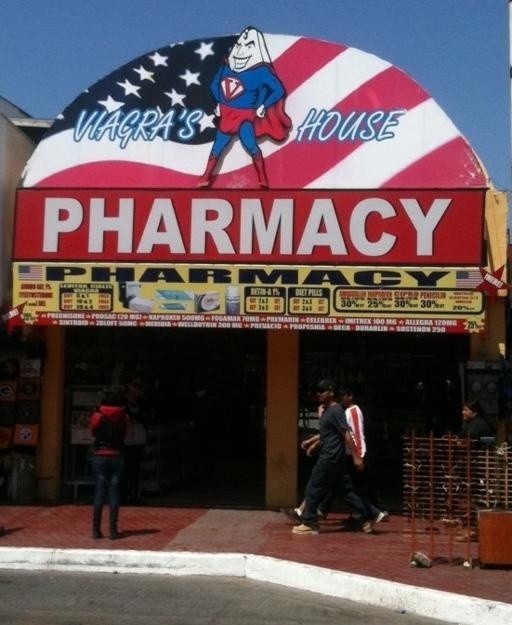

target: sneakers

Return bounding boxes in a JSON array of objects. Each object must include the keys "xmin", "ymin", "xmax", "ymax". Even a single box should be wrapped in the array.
[
  {"xmin": 280, "ymin": 505, "xmax": 304, "ymax": 521},
  {"xmin": 292, "ymin": 523, "xmax": 320, "ymax": 536},
  {"xmin": 375, "ymin": 509, "xmax": 389, "ymax": 524},
  {"xmin": 361, "ymin": 521, "xmax": 373, "ymax": 534}
]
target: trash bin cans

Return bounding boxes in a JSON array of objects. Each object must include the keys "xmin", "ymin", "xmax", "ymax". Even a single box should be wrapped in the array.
[
  {"xmin": 477, "ymin": 510, "xmax": 511, "ymax": 569},
  {"xmin": 2, "ymin": 445, "xmax": 38, "ymax": 502}
]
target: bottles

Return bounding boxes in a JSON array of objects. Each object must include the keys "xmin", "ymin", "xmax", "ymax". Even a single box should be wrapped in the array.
[{"xmin": 225, "ymin": 284, "xmax": 241, "ymax": 315}]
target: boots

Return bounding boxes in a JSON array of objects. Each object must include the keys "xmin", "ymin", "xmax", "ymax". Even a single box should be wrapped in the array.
[
  {"xmin": 110, "ymin": 509, "xmax": 123, "ymax": 539},
  {"xmin": 93, "ymin": 510, "xmax": 102, "ymax": 538}
]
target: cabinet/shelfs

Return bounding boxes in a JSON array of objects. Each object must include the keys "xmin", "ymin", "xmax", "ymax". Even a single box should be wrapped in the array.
[{"xmin": 63, "ymin": 385, "xmax": 180, "ymax": 499}]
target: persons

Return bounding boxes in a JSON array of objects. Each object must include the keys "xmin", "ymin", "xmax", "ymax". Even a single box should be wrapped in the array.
[
  {"xmin": 88, "ymin": 390, "xmax": 135, "ymax": 540},
  {"xmin": 456, "ymin": 400, "xmax": 490, "ymax": 542},
  {"xmin": 284, "ymin": 378, "xmax": 389, "ymax": 536}
]
